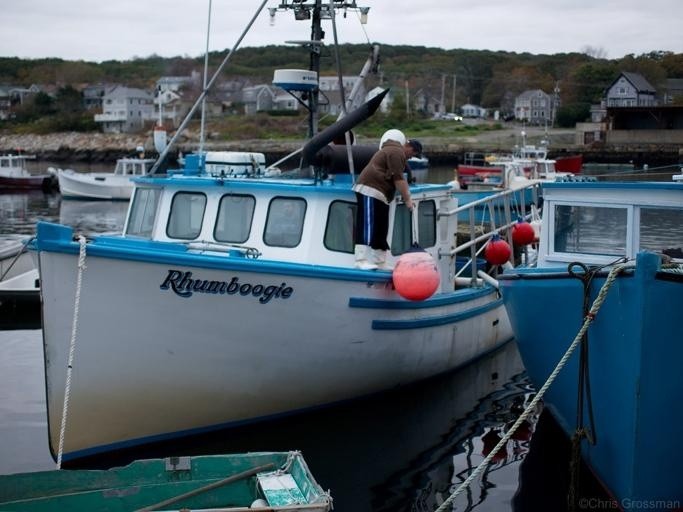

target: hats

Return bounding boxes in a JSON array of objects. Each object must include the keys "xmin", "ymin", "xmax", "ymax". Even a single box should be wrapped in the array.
[{"xmin": 408, "ymin": 138, "xmax": 422, "ymax": 160}]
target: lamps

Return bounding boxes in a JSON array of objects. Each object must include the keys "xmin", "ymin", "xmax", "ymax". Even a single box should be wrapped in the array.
[
  {"xmin": 359, "ymin": 7, "xmax": 369, "ymax": 24},
  {"xmin": 269, "ymin": 8, "xmax": 277, "ymax": 27}
]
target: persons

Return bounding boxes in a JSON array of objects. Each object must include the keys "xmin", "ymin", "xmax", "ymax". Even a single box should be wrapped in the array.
[{"xmin": 348, "ymin": 137, "xmax": 421, "ymax": 274}]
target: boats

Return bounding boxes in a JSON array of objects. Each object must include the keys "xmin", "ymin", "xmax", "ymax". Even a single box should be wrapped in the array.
[
  {"xmin": 1, "ymin": 155, "xmax": 54, "ymax": 192},
  {"xmin": 1, "ymin": 451, "xmax": 333, "ymax": 510},
  {"xmin": 36, "ymin": 1, "xmax": 583, "ymax": 465},
  {"xmin": 479, "ymin": 176, "xmax": 683, "ymax": 512}
]
